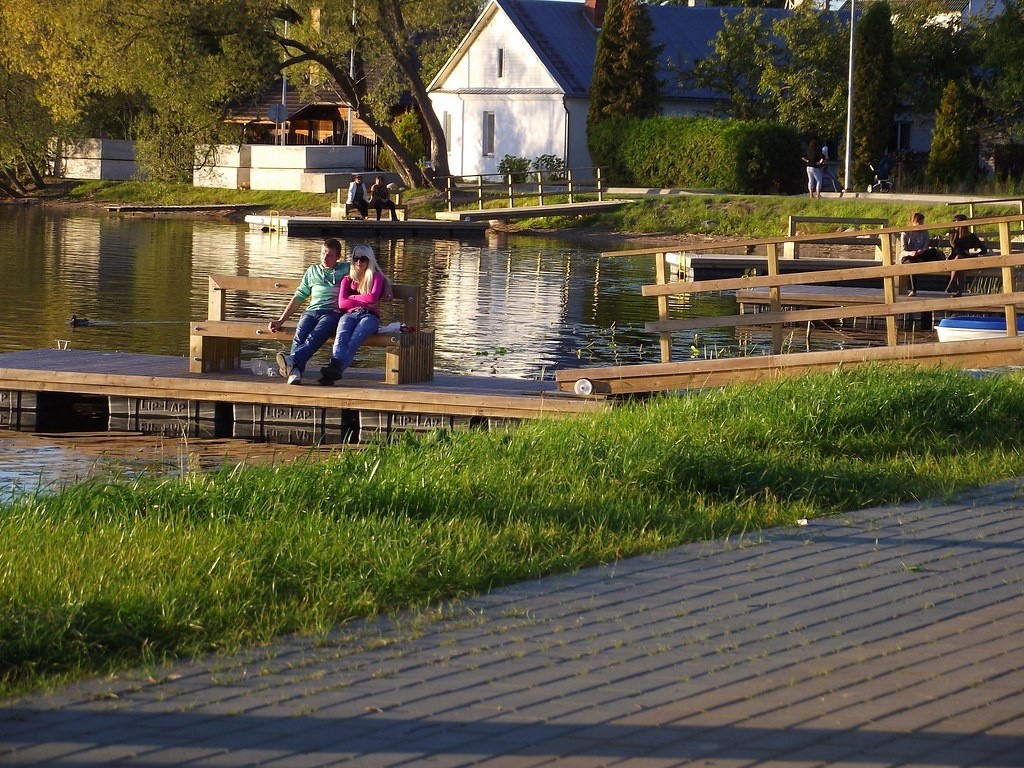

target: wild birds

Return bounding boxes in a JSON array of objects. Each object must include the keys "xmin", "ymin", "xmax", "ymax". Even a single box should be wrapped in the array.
[{"xmin": 68, "ymin": 314, "xmax": 90, "ymax": 327}]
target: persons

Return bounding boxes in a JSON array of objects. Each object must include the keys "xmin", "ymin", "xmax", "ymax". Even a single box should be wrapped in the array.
[
  {"xmin": 944, "ymin": 214, "xmax": 986, "ymax": 297},
  {"xmin": 368, "ymin": 176, "xmax": 401, "ymax": 222},
  {"xmin": 811, "ymin": 141, "xmax": 844, "ymax": 196},
  {"xmin": 801, "ymin": 139, "xmax": 823, "ymax": 199},
  {"xmin": 268, "ymin": 238, "xmax": 393, "ymax": 386},
  {"xmin": 899, "ymin": 213, "xmax": 962, "ymax": 298},
  {"xmin": 346, "ymin": 175, "xmax": 370, "ymax": 221},
  {"xmin": 317, "ymin": 243, "xmax": 383, "ymax": 386}
]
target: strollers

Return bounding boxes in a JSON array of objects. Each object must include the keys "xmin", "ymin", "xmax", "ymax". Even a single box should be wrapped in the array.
[{"xmin": 866, "ymin": 156, "xmax": 896, "ymax": 194}]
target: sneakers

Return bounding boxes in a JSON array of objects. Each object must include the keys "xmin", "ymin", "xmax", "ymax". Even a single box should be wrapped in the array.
[{"xmin": 276, "ymin": 352, "xmax": 302, "ymax": 384}]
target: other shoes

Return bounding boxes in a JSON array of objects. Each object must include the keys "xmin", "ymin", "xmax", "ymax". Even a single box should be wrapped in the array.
[{"xmin": 317, "ymin": 358, "xmax": 343, "ymax": 385}]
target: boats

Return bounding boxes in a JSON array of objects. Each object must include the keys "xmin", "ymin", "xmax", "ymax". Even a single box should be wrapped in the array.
[{"xmin": 933, "ymin": 315, "xmax": 1024, "ymax": 376}]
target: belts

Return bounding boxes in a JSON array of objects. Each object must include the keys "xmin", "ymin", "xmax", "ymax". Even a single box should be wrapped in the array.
[{"xmin": 351, "ymin": 307, "xmax": 380, "ymax": 319}]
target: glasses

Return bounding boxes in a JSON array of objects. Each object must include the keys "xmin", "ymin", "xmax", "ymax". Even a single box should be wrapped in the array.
[{"xmin": 353, "ymin": 255, "xmax": 368, "ymax": 262}]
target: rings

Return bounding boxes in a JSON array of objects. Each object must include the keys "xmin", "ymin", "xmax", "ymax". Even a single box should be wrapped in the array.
[{"xmin": 388, "ymin": 294, "xmax": 392, "ymax": 296}]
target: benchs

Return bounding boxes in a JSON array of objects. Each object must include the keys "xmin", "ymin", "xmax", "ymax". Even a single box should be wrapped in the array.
[
  {"xmin": 891, "ymin": 234, "xmax": 1024, "ymax": 297},
  {"xmin": 189, "ymin": 274, "xmax": 436, "ymax": 385},
  {"xmin": 330, "ymin": 188, "xmax": 408, "ymax": 222}
]
